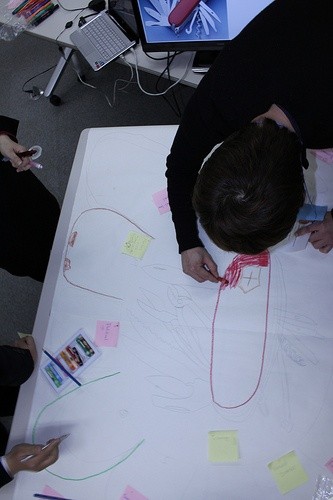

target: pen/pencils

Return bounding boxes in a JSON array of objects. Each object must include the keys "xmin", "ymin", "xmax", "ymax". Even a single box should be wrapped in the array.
[
  {"xmin": 42, "ymin": 348, "xmax": 81, "ymax": 386},
  {"xmin": 30, "ymin": 162, "xmax": 43, "ymax": 169},
  {"xmin": 2, "ymin": 149, "xmax": 37, "ymax": 162},
  {"xmin": 19, "ymin": 433, "xmax": 70, "ymax": 462}
]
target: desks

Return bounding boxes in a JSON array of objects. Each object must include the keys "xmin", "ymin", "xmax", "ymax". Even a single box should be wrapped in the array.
[
  {"xmin": 0, "ymin": 0, "xmax": 211, "ymax": 106},
  {"xmin": 0, "ymin": 125, "xmax": 333, "ymax": 500}
]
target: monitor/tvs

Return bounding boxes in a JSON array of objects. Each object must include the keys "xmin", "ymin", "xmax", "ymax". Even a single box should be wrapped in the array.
[{"xmin": 136, "ymin": 0, "xmax": 277, "ymax": 74}]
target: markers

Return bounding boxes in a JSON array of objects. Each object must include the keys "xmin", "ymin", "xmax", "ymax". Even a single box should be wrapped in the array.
[{"xmin": 11, "ymin": 0, "xmax": 59, "ymax": 26}]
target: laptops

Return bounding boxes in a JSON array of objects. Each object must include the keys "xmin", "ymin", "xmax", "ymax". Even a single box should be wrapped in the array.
[{"xmin": 69, "ymin": 0, "xmax": 139, "ymax": 72}]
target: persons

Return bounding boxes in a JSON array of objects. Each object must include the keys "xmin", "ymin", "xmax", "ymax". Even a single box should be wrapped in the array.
[
  {"xmin": 0, "ymin": 336, "xmax": 59, "ymax": 488},
  {"xmin": 165, "ymin": 0, "xmax": 333, "ymax": 284},
  {"xmin": 0, "ymin": 115, "xmax": 61, "ymax": 283}
]
